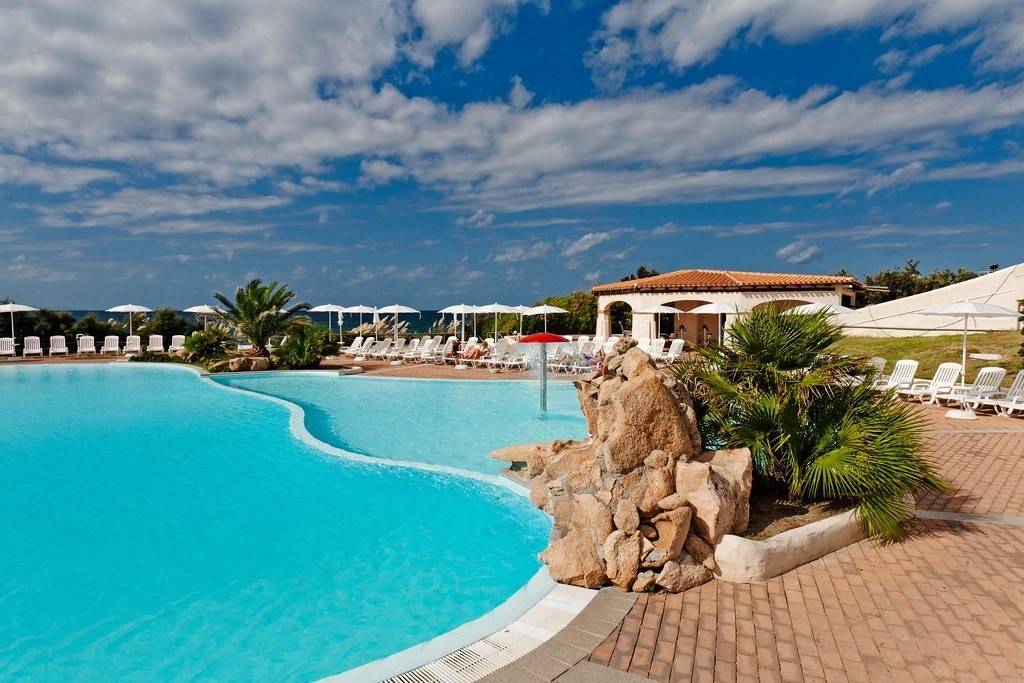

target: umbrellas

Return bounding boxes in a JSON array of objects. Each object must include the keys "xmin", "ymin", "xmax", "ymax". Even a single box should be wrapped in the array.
[
  {"xmin": 105, "ymin": 304, "xmax": 155, "ymax": 357},
  {"xmin": 279, "ymin": 309, "xmax": 289, "ymax": 313},
  {"xmin": 473, "ymin": 303, "xmax": 523, "ymax": 373},
  {"xmin": 0, "ymin": 302, "xmax": 39, "ymax": 361},
  {"xmin": 438, "ymin": 303, "xmax": 487, "ymax": 370},
  {"xmin": 631, "ymin": 304, "xmax": 684, "ymax": 340},
  {"xmin": 520, "ymin": 304, "xmax": 570, "ymax": 333},
  {"xmin": 511, "ymin": 304, "xmax": 532, "ymax": 340},
  {"xmin": 341, "ymin": 304, "xmax": 380, "ymax": 361},
  {"xmin": 914, "ymin": 300, "xmax": 1024, "ymax": 420},
  {"xmin": 375, "ymin": 303, "xmax": 421, "ymax": 365},
  {"xmin": 777, "ymin": 301, "xmax": 854, "ymax": 345},
  {"xmin": 686, "ymin": 301, "xmax": 753, "ymax": 345},
  {"xmin": 307, "ymin": 303, "xmax": 347, "ymax": 359},
  {"xmin": 182, "ymin": 304, "xmax": 228, "ymax": 331}
]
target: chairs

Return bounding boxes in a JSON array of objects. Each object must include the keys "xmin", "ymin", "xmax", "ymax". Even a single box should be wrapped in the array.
[
  {"xmin": 100, "ymin": 336, "xmax": 122, "ymax": 356},
  {"xmin": 0, "ymin": 337, "xmax": 16, "ymax": 357},
  {"xmin": 77, "ymin": 336, "xmax": 96, "ymax": 356},
  {"xmin": 123, "ymin": 336, "xmax": 143, "ymax": 354},
  {"xmin": 22, "ymin": 337, "xmax": 44, "ymax": 359},
  {"xmin": 147, "ymin": 335, "xmax": 165, "ymax": 356},
  {"xmin": 169, "ymin": 335, "xmax": 187, "ymax": 354},
  {"xmin": 346, "ymin": 338, "xmax": 1024, "ymax": 416},
  {"xmin": 49, "ymin": 336, "xmax": 69, "ymax": 357}
]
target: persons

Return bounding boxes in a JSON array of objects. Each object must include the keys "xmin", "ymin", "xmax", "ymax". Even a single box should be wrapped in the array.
[
  {"xmin": 443, "ymin": 340, "xmax": 459, "ymax": 366},
  {"xmin": 584, "ymin": 346, "xmax": 606, "ymax": 366},
  {"xmin": 556, "ymin": 351, "xmax": 587, "ymax": 370},
  {"xmin": 466, "ymin": 341, "xmax": 490, "ymax": 366},
  {"xmin": 513, "ymin": 330, "xmax": 520, "ymax": 340}
]
